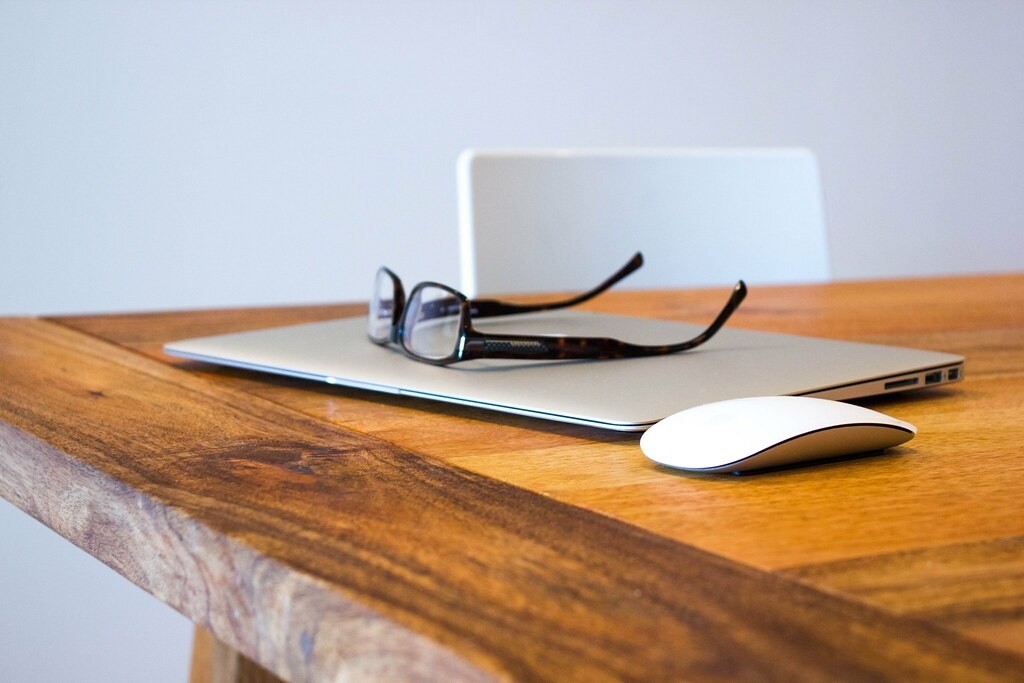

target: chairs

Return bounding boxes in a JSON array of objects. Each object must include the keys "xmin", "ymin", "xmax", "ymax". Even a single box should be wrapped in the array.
[{"xmin": 459, "ymin": 148, "xmax": 829, "ymax": 292}]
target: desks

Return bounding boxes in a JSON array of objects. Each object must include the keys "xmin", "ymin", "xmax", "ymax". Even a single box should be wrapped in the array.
[{"xmin": 0, "ymin": 274, "xmax": 1024, "ymax": 683}]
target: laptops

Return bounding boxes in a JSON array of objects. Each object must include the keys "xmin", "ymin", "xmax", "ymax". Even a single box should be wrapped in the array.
[{"xmin": 163, "ymin": 305, "xmax": 966, "ymax": 432}]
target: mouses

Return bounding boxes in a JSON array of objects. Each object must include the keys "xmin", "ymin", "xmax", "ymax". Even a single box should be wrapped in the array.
[{"xmin": 639, "ymin": 394, "xmax": 917, "ymax": 475}]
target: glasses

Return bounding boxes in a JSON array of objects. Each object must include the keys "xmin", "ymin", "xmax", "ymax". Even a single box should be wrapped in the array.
[{"xmin": 364, "ymin": 252, "xmax": 750, "ymax": 366}]
